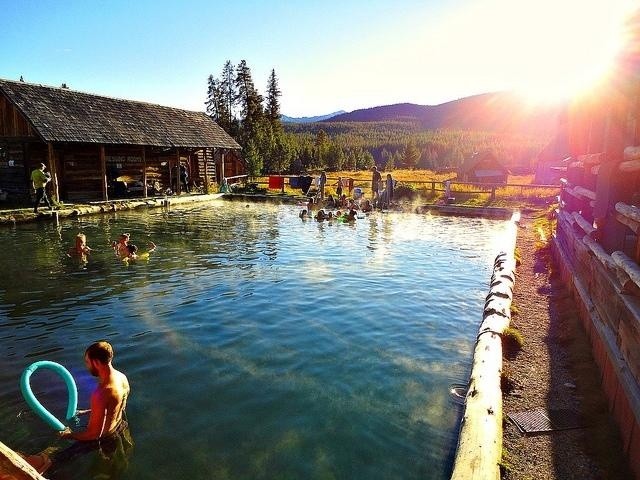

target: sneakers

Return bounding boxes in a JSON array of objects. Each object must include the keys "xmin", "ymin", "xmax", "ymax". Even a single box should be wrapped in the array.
[
  {"xmin": 34, "ymin": 211, "xmax": 38, "ymax": 213},
  {"xmin": 49, "ymin": 207, "xmax": 53, "ymax": 210}
]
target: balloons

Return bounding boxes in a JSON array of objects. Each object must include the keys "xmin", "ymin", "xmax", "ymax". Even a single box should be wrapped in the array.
[{"xmin": 20, "ymin": 360, "xmax": 78, "ymax": 434}]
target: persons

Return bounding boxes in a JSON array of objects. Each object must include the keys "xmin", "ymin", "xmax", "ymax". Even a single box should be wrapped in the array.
[
  {"xmin": 170, "ymin": 164, "xmax": 178, "ymax": 195},
  {"xmin": 110, "ymin": 232, "xmax": 130, "ymax": 257},
  {"xmin": 30, "ymin": 162, "xmax": 54, "ymax": 213},
  {"xmin": 64, "ymin": 233, "xmax": 99, "ymax": 260},
  {"xmin": 299, "ymin": 165, "xmax": 398, "ymax": 227},
  {"xmin": 179, "ymin": 162, "xmax": 191, "ymax": 195},
  {"xmin": 122, "ymin": 241, "xmax": 157, "ymax": 265},
  {"xmin": 55, "ymin": 341, "xmax": 134, "ymax": 476}
]
